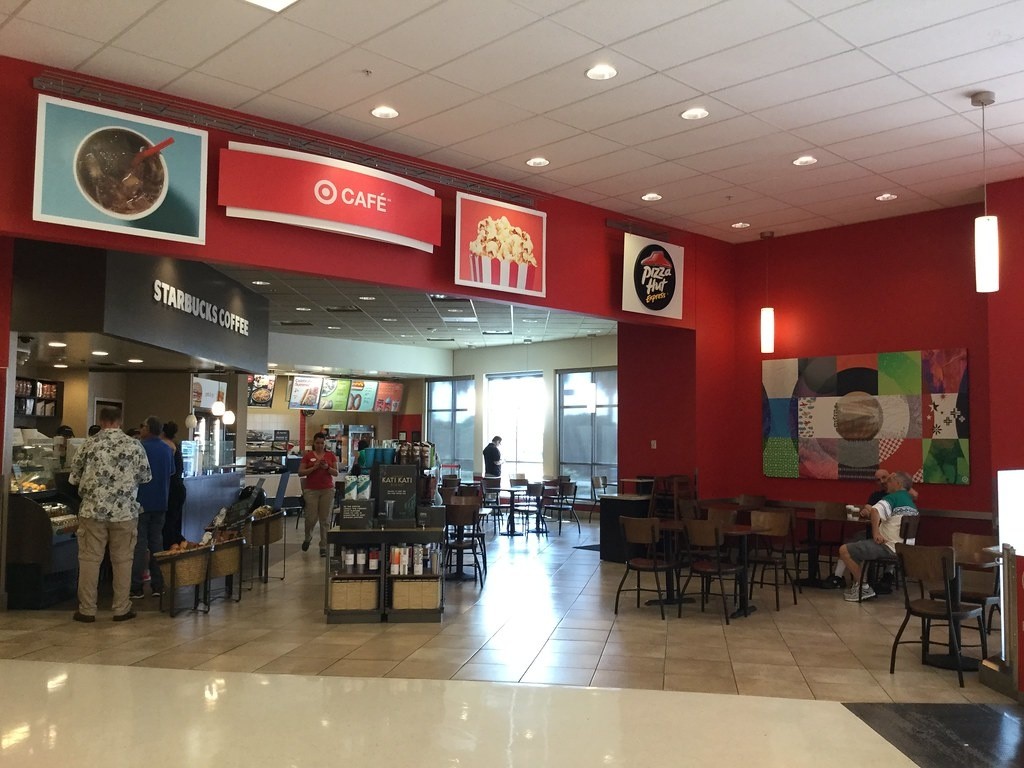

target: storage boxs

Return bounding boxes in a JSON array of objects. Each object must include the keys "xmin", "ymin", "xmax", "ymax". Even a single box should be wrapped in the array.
[{"xmin": 340, "ymin": 473, "xmax": 445, "ymax": 575}]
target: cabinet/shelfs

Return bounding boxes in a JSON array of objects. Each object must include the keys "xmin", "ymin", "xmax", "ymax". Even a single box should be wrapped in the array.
[
  {"xmin": 14, "ymin": 377, "xmax": 63, "ymax": 437},
  {"xmin": 598, "ymin": 477, "xmax": 654, "ymax": 564},
  {"xmin": 324, "ymin": 468, "xmax": 445, "ymax": 623}
]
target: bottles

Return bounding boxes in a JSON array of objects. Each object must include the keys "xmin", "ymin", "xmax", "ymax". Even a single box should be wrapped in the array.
[
  {"xmin": 341, "ymin": 543, "xmax": 441, "ymax": 576},
  {"xmin": 384, "ymin": 398, "xmax": 391, "ymax": 411}
]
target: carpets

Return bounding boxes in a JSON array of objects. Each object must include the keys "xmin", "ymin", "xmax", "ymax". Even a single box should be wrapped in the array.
[{"xmin": 574, "ymin": 545, "xmax": 600, "ymax": 552}]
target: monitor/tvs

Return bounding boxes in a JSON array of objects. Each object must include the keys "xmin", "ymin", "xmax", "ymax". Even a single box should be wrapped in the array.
[{"xmin": 66, "ymin": 438, "xmax": 86, "ymax": 467}]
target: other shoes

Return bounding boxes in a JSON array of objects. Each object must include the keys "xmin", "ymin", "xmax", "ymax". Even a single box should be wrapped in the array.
[
  {"xmin": 74, "ymin": 612, "xmax": 95, "ymax": 623},
  {"xmin": 129, "ymin": 591, "xmax": 144, "ymax": 599},
  {"xmin": 153, "ymin": 587, "xmax": 165, "ymax": 596},
  {"xmin": 302, "ymin": 540, "xmax": 310, "ymax": 552},
  {"xmin": 878, "ymin": 571, "xmax": 895, "ymax": 591},
  {"xmin": 320, "ymin": 549, "xmax": 327, "ymax": 557},
  {"xmin": 113, "ymin": 609, "xmax": 137, "ymax": 621},
  {"xmin": 142, "ymin": 570, "xmax": 151, "ymax": 582},
  {"xmin": 820, "ymin": 573, "xmax": 845, "ymax": 589}
]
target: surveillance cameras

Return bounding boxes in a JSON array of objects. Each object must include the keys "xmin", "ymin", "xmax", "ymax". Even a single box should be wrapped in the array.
[{"xmin": 18, "ymin": 336, "xmax": 34, "ymax": 342}]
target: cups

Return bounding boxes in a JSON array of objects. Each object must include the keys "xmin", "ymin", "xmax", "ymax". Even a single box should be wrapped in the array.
[
  {"xmin": 73, "ymin": 126, "xmax": 169, "ymax": 221},
  {"xmin": 852, "ymin": 507, "xmax": 860, "ymax": 521},
  {"xmin": 846, "ymin": 505, "xmax": 854, "ymax": 520},
  {"xmin": 390, "ymin": 438, "xmax": 431, "ymax": 456},
  {"xmin": 376, "ymin": 399, "xmax": 384, "ymax": 411},
  {"xmin": 365, "ymin": 448, "xmax": 397, "ymax": 467},
  {"xmin": 392, "ymin": 400, "xmax": 399, "ymax": 411}
]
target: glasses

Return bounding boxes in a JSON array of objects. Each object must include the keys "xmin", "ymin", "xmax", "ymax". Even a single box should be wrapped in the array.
[{"xmin": 139, "ymin": 423, "xmax": 148, "ymax": 430}]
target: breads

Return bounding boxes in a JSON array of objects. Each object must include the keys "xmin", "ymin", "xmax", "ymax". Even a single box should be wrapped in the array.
[
  {"xmin": 347, "ymin": 393, "xmax": 361, "ymax": 410},
  {"xmin": 21, "ymin": 481, "xmax": 47, "ymax": 491}
]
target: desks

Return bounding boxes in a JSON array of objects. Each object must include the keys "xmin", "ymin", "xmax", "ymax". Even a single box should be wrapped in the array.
[
  {"xmin": 603, "ymin": 482, "xmax": 618, "ymax": 488},
  {"xmin": 644, "ymin": 519, "xmax": 773, "ymax": 618},
  {"xmin": 697, "ymin": 500, "xmax": 746, "ymax": 562},
  {"xmin": 460, "ymin": 480, "xmax": 481, "ymax": 487},
  {"xmin": 486, "ymin": 484, "xmax": 559, "ymax": 536},
  {"xmin": 925, "ymin": 552, "xmax": 998, "ymax": 672},
  {"xmin": 792, "ymin": 511, "xmax": 872, "ymax": 589},
  {"xmin": 444, "ymin": 508, "xmax": 492, "ymax": 582}
]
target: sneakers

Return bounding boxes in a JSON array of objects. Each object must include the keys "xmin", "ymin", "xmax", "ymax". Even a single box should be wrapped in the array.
[{"xmin": 843, "ymin": 581, "xmax": 876, "ymax": 602}]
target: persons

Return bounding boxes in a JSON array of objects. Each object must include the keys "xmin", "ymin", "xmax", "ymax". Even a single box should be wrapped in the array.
[
  {"xmin": 354, "ymin": 439, "xmax": 370, "ymax": 475},
  {"xmin": 68, "ymin": 406, "xmax": 153, "ymax": 623},
  {"xmin": 297, "ymin": 432, "xmax": 338, "ymax": 556},
  {"xmin": 56, "ymin": 425, "xmax": 75, "ymax": 439},
  {"xmin": 127, "ymin": 416, "xmax": 186, "ymax": 598},
  {"xmin": 88, "ymin": 425, "xmax": 101, "ymax": 437},
  {"xmin": 483, "ymin": 436, "xmax": 505, "ymax": 500},
  {"xmin": 820, "ymin": 469, "xmax": 919, "ymax": 602}
]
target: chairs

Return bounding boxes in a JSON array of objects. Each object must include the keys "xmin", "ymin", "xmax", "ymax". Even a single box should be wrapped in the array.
[
  {"xmin": 438, "ymin": 474, "xmax": 607, "ymax": 588},
  {"xmin": 613, "ymin": 493, "xmax": 999, "ymax": 690}
]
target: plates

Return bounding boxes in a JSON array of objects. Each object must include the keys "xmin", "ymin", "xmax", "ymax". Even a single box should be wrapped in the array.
[
  {"xmin": 321, "ymin": 380, "xmax": 338, "ymax": 396},
  {"xmin": 252, "ymin": 387, "xmax": 272, "ymax": 403}
]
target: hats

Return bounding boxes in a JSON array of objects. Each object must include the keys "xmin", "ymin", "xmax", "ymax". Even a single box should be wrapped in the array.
[
  {"xmin": 126, "ymin": 428, "xmax": 140, "ymax": 437},
  {"xmin": 56, "ymin": 425, "xmax": 75, "ymax": 437},
  {"xmin": 87, "ymin": 425, "xmax": 101, "ymax": 436}
]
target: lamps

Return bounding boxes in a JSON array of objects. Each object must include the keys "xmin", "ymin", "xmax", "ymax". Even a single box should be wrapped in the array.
[
  {"xmin": 185, "ymin": 364, "xmax": 235, "ymax": 429},
  {"xmin": 972, "ymin": 91, "xmax": 1000, "ymax": 293},
  {"xmin": 760, "ymin": 231, "xmax": 775, "ymax": 353}
]
